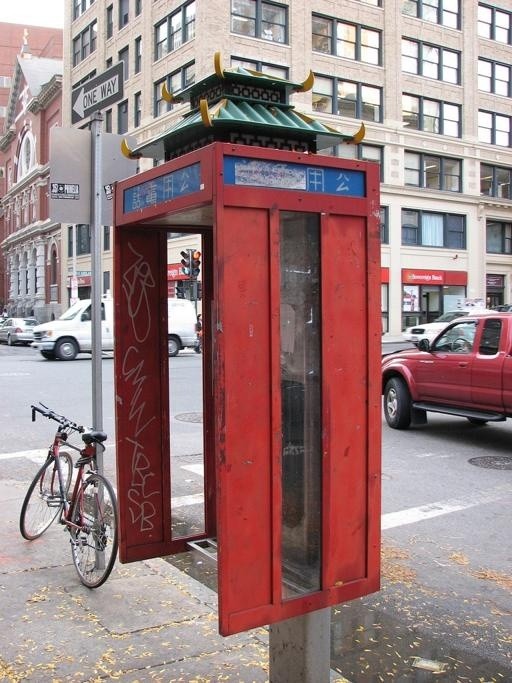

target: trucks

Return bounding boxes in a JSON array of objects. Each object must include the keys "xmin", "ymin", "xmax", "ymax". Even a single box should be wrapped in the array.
[{"xmin": 31, "ymin": 292, "xmax": 200, "ymax": 359}]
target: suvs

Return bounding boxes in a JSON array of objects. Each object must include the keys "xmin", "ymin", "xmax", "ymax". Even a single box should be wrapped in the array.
[{"xmin": 404, "ymin": 305, "xmax": 502, "ymax": 352}]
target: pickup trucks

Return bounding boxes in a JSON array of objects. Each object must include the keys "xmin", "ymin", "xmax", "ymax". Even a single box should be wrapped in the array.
[{"xmin": 380, "ymin": 311, "xmax": 511, "ymax": 435}]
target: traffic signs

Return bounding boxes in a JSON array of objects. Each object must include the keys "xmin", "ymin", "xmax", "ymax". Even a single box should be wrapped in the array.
[{"xmin": 69, "ymin": 58, "xmax": 125, "ymax": 125}]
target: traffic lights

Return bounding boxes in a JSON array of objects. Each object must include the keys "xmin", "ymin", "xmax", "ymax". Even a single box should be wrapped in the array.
[
  {"xmin": 191, "ymin": 247, "xmax": 200, "ymax": 278},
  {"xmin": 178, "ymin": 248, "xmax": 189, "ymax": 278},
  {"xmin": 174, "ymin": 286, "xmax": 185, "ymax": 297}
]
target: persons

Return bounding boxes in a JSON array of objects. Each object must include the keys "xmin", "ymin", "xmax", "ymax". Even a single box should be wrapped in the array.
[
  {"xmin": 192, "ymin": 313, "xmax": 204, "ymax": 354},
  {"xmin": 0, "ymin": 307, "xmax": 10, "ymax": 317}
]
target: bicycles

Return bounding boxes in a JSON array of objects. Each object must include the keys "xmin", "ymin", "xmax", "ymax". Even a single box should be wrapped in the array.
[{"xmin": 16, "ymin": 401, "xmax": 118, "ymax": 591}]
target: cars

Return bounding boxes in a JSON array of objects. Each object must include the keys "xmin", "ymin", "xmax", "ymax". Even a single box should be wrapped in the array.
[{"xmin": 0, "ymin": 314, "xmax": 41, "ymax": 347}]
